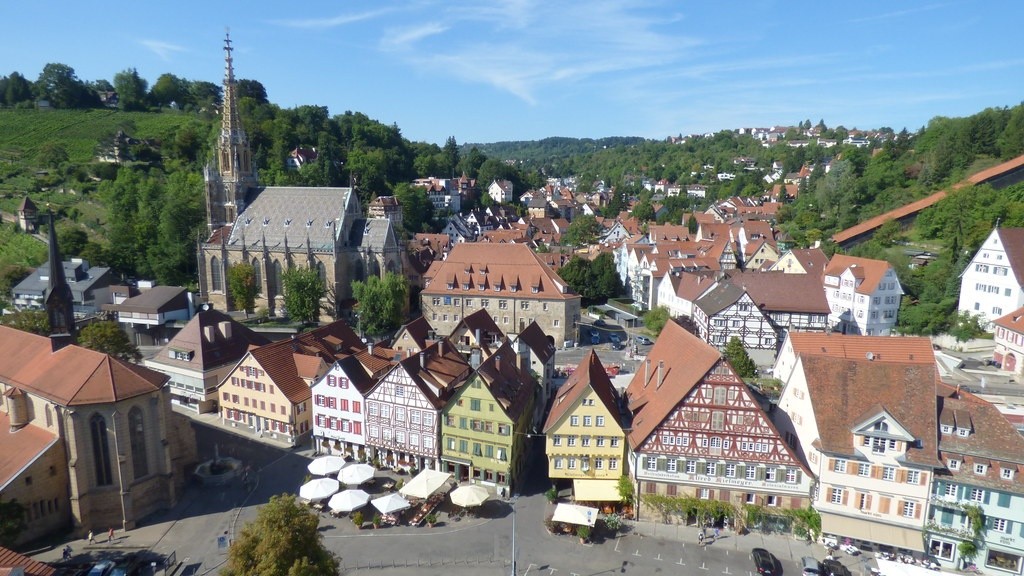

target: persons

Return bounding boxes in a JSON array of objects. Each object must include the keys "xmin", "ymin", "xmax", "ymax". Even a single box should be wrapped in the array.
[
  {"xmin": 88, "ymin": 529, "xmax": 97, "ymax": 545},
  {"xmin": 108, "ymin": 526, "xmax": 113, "ymax": 542},
  {"xmin": 697, "ymin": 527, "xmax": 718, "ymax": 544},
  {"xmin": 63, "ymin": 544, "xmax": 72, "ymax": 563},
  {"xmin": 827, "ymin": 549, "xmax": 835, "ymax": 560}
]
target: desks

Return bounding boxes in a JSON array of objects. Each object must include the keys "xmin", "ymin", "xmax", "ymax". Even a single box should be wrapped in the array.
[
  {"xmin": 408, "ymin": 495, "xmax": 441, "ymax": 526},
  {"xmin": 300, "ymin": 500, "xmax": 340, "ymax": 518}
]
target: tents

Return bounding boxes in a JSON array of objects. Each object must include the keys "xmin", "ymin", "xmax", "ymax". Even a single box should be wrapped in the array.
[{"xmin": 300, "ymin": 456, "xmax": 599, "ymax": 534}]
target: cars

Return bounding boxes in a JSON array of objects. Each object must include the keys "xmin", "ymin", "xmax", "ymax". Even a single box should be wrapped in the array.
[
  {"xmin": 612, "ymin": 341, "xmax": 622, "ymax": 350},
  {"xmin": 752, "ymin": 548, "xmax": 779, "ymax": 576},
  {"xmin": 55, "ymin": 562, "xmax": 94, "ymax": 576},
  {"xmin": 635, "ymin": 335, "xmax": 651, "ymax": 345},
  {"xmin": 593, "ymin": 319, "xmax": 606, "ymax": 328},
  {"xmin": 589, "ymin": 328, "xmax": 600, "ymax": 337},
  {"xmin": 108, "ymin": 557, "xmax": 138, "ymax": 576},
  {"xmin": 591, "ymin": 335, "xmax": 600, "ymax": 345},
  {"xmin": 86, "ymin": 559, "xmax": 116, "ymax": 576},
  {"xmin": 609, "ymin": 333, "xmax": 621, "ymax": 343},
  {"xmin": 801, "ymin": 556, "xmax": 823, "ymax": 576},
  {"xmin": 822, "ymin": 559, "xmax": 846, "ymax": 576}
]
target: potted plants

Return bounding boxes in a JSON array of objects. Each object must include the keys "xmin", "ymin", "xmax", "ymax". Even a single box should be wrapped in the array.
[
  {"xmin": 349, "ymin": 515, "xmax": 353, "ymax": 522},
  {"xmin": 426, "ymin": 513, "xmax": 436, "ymax": 528},
  {"xmin": 353, "ymin": 510, "xmax": 363, "ymax": 529},
  {"xmin": 546, "ymin": 488, "xmax": 557, "ymax": 505},
  {"xmin": 577, "ymin": 526, "xmax": 590, "ymax": 544},
  {"xmin": 373, "ymin": 513, "xmax": 381, "ymax": 530}
]
